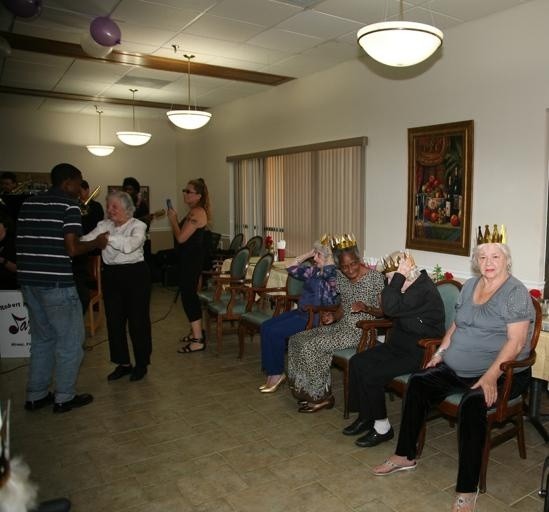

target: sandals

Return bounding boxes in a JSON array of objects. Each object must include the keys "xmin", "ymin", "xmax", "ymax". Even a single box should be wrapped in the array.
[
  {"xmin": 178, "ymin": 338, "xmax": 206, "ymax": 353},
  {"xmin": 180, "ymin": 329, "xmax": 206, "ymax": 342}
]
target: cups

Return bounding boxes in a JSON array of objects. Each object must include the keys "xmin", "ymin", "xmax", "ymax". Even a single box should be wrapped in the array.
[
  {"xmin": 538, "ymin": 298, "xmax": 549, "ymax": 318},
  {"xmin": 278, "ymin": 248, "xmax": 285, "ymax": 260}
]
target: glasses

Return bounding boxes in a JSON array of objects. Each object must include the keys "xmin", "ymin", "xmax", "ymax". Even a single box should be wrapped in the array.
[{"xmin": 182, "ymin": 189, "xmax": 194, "ymax": 194}]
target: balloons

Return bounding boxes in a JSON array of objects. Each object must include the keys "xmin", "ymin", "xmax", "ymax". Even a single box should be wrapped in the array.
[
  {"xmin": 89, "ymin": 17, "xmax": 122, "ymax": 47},
  {"xmin": 0, "ymin": 37, "xmax": 12, "ymax": 59},
  {"xmin": 80, "ymin": 31, "xmax": 113, "ymax": 58},
  {"xmin": 1, "ymin": 0, "xmax": 43, "ymax": 18}
]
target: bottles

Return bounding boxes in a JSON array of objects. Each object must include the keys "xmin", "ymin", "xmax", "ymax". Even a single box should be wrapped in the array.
[
  {"xmin": 446, "ymin": 167, "xmax": 462, "ymax": 219},
  {"xmin": 413, "ymin": 195, "xmax": 423, "ymax": 220}
]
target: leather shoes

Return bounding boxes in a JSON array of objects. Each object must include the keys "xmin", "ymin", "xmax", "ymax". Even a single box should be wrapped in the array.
[
  {"xmin": 108, "ymin": 364, "xmax": 132, "ymax": 380},
  {"xmin": 130, "ymin": 366, "xmax": 147, "ymax": 381},
  {"xmin": 24, "ymin": 392, "xmax": 54, "ymax": 410},
  {"xmin": 53, "ymin": 394, "xmax": 92, "ymax": 413},
  {"xmin": 259, "ymin": 371, "xmax": 480, "ymax": 512}
]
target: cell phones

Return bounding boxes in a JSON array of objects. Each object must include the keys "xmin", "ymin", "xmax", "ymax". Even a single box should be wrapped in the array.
[{"xmin": 167, "ymin": 199, "xmax": 173, "ymax": 210}]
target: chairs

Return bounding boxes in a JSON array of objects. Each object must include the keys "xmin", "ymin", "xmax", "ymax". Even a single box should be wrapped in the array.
[{"xmin": 80, "ymin": 255, "xmax": 107, "ymax": 335}]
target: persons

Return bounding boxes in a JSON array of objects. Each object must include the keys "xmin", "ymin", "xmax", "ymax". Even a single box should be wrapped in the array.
[
  {"xmin": 13, "ymin": 163, "xmax": 110, "ymax": 414},
  {"xmin": 0, "ymin": 174, "xmax": 153, "ymax": 314},
  {"xmin": 258, "ymin": 238, "xmax": 339, "ymax": 394},
  {"xmin": 370, "ymin": 235, "xmax": 537, "ymax": 512},
  {"xmin": 78, "ymin": 192, "xmax": 153, "ymax": 382},
  {"xmin": 344, "ymin": 251, "xmax": 445, "ymax": 448},
  {"xmin": 285, "ymin": 244, "xmax": 387, "ymax": 413},
  {"xmin": 168, "ymin": 180, "xmax": 209, "ymax": 353}
]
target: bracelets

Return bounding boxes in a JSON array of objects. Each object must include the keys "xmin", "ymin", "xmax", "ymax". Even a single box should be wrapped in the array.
[{"xmin": 431, "ymin": 348, "xmax": 445, "ymax": 357}]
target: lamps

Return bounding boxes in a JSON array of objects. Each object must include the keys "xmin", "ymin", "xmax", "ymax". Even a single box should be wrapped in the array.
[
  {"xmin": 86, "ymin": 102, "xmax": 116, "ymax": 156},
  {"xmin": 116, "ymin": 88, "xmax": 152, "ymax": 146},
  {"xmin": 166, "ymin": 54, "xmax": 212, "ymax": 129},
  {"xmin": 357, "ymin": 0, "xmax": 445, "ymax": 68}
]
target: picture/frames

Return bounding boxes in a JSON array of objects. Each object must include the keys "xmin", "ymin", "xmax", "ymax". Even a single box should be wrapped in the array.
[
  {"xmin": 108, "ymin": 186, "xmax": 149, "ymax": 213},
  {"xmin": 405, "ymin": 119, "xmax": 474, "ymax": 257}
]
